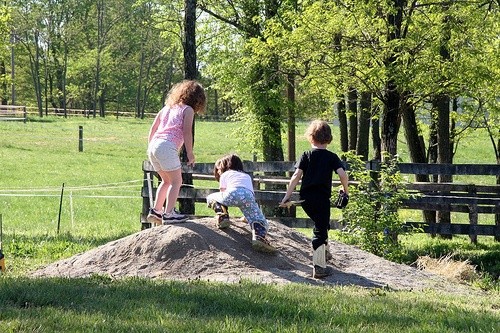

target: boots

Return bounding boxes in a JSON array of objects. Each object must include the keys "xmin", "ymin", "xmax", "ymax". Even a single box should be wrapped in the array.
[
  {"xmin": 250, "ymin": 220, "xmax": 279, "ymax": 256},
  {"xmin": 208, "ymin": 199, "xmax": 230, "ymax": 228}
]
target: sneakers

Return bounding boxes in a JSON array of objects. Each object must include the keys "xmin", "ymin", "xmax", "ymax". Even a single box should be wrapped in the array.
[{"xmin": 147, "ymin": 207, "xmax": 189, "ymax": 225}]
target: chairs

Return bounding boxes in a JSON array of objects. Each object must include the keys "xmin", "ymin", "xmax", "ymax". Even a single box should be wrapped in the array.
[{"xmin": 0, "ymin": 105, "xmax": 29, "ymax": 124}]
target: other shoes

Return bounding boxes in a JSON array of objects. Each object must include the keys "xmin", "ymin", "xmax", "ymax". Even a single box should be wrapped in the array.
[
  {"xmin": 324, "ymin": 251, "xmax": 332, "ymax": 260},
  {"xmin": 313, "ymin": 266, "xmax": 333, "ymax": 278}
]
